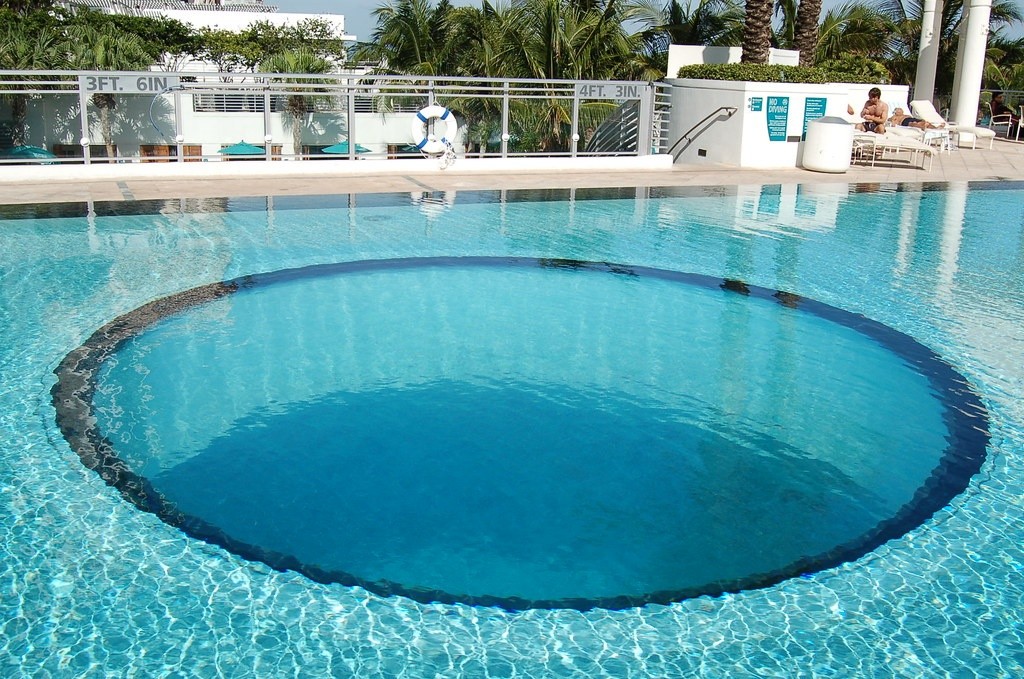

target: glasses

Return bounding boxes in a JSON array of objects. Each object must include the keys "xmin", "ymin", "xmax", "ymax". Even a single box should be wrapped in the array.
[{"xmin": 997, "ymin": 96, "xmax": 1002, "ymax": 98}]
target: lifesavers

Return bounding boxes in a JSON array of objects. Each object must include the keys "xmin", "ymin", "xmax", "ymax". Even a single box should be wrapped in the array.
[{"xmin": 411, "ymin": 105, "xmax": 458, "ymax": 154}]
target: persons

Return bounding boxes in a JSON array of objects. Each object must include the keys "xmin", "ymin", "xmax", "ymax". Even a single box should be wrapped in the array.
[
  {"xmin": 990, "ymin": 91, "xmax": 1020, "ymax": 136},
  {"xmin": 848, "ymin": 87, "xmax": 889, "ymax": 134},
  {"xmin": 888, "ymin": 108, "xmax": 944, "ymax": 131}
]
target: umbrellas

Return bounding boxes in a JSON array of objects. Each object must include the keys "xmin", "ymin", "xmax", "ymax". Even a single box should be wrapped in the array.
[
  {"xmin": 322, "ymin": 139, "xmax": 372, "ymax": 154},
  {"xmin": 217, "ymin": 140, "xmax": 265, "ymax": 154},
  {"xmin": 403, "ymin": 146, "xmax": 424, "ymax": 152},
  {"xmin": 0, "ymin": 146, "xmax": 60, "ymax": 166}
]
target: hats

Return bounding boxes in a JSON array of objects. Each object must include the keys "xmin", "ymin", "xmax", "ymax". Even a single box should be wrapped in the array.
[{"xmin": 992, "ymin": 92, "xmax": 1003, "ymax": 100}]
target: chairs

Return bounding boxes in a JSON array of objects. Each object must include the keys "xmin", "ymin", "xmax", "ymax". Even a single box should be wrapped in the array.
[{"xmin": 850, "ymin": 102, "xmax": 1024, "ymax": 173}]
